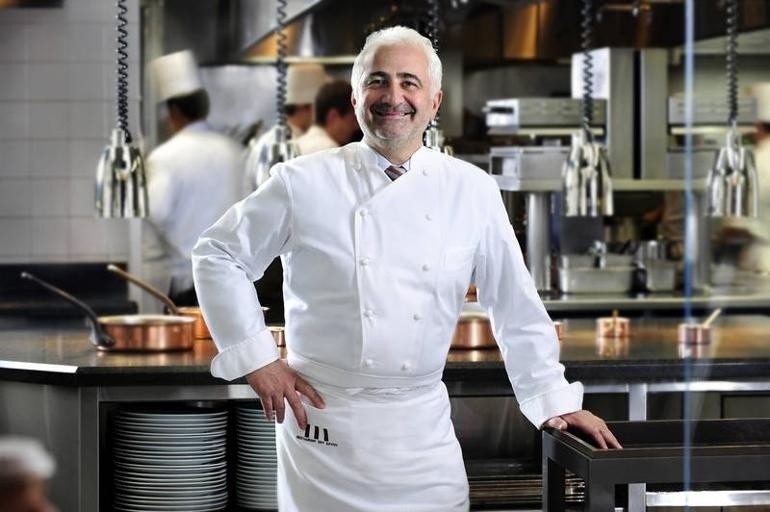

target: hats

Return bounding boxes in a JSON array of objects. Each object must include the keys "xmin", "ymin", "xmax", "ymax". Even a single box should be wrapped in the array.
[
  {"xmin": 285, "ymin": 64, "xmax": 326, "ymax": 107},
  {"xmin": 152, "ymin": 50, "xmax": 202, "ymax": 103}
]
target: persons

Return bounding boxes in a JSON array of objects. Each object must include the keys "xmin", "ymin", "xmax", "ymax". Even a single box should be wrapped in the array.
[
  {"xmin": 190, "ymin": 25, "xmax": 624, "ymax": 512},
  {"xmin": 287, "ymin": 79, "xmax": 361, "ymax": 157},
  {"xmin": 137, "ymin": 49, "xmax": 248, "ymax": 308},
  {"xmin": 247, "ymin": 64, "xmax": 332, "ymax": 187}
]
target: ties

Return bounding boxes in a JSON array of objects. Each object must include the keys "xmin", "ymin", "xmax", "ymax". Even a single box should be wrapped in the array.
[{"xmin": 385, "ymin": 166, "xmax": 406, "ymax": 181}]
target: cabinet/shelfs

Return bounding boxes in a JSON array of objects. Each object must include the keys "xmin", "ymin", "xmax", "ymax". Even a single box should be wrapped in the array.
[{"xmin": 1, "ymin": 317, "xmax": 770, "ymax": 512}]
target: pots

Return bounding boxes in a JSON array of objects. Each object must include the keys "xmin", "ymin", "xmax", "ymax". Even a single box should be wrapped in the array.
[
  {"xmin": 18, "ymin": 270, "xmax": 196, "ymax": 352},
  {"xmin": 596, "ymin": 308, "xmax": 630, "ymax": 337},
  {"xmin": 107, "ymin": 262, "xmax": 269, "ymax": 339},
  {"xmin": 678, "ymin": 308, "xmax": 721, "ymax": 344},
  {"xmin": 450, "ymin": 315, "xmax": 501, "ymax": 350}
]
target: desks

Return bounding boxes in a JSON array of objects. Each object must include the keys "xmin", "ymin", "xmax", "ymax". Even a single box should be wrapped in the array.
[{"xmin": 540, "ymin": 419, "xmax": 770, "ymax": 512}]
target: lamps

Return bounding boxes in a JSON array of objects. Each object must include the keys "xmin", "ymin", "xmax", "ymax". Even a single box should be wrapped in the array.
[
  {"xmin": 93, "ymin": 0, "xmax": 148, "ymax": 221},
  {"xmin": 423, "ymin": 0, "xmax": 454, "ymax": 160},
  {"xmin": 704, "ymin": 1, "xmax": 757, "ymax": 220},
  {"xmin": 561, "ymin": 0, "xmax": 614, "ymax": 220},
  {"xmin": 240, "ymin": 0, "xmax": 302, "ymax": 198}
]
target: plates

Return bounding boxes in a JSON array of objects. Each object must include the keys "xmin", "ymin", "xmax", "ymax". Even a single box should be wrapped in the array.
[
  {"xmin": 236, "ymin": 407, "xmax": 277, "ymax": 510},
  {"xmin": 115, "ymin": 409, "xmax": 227, "ymax": 512}
]
